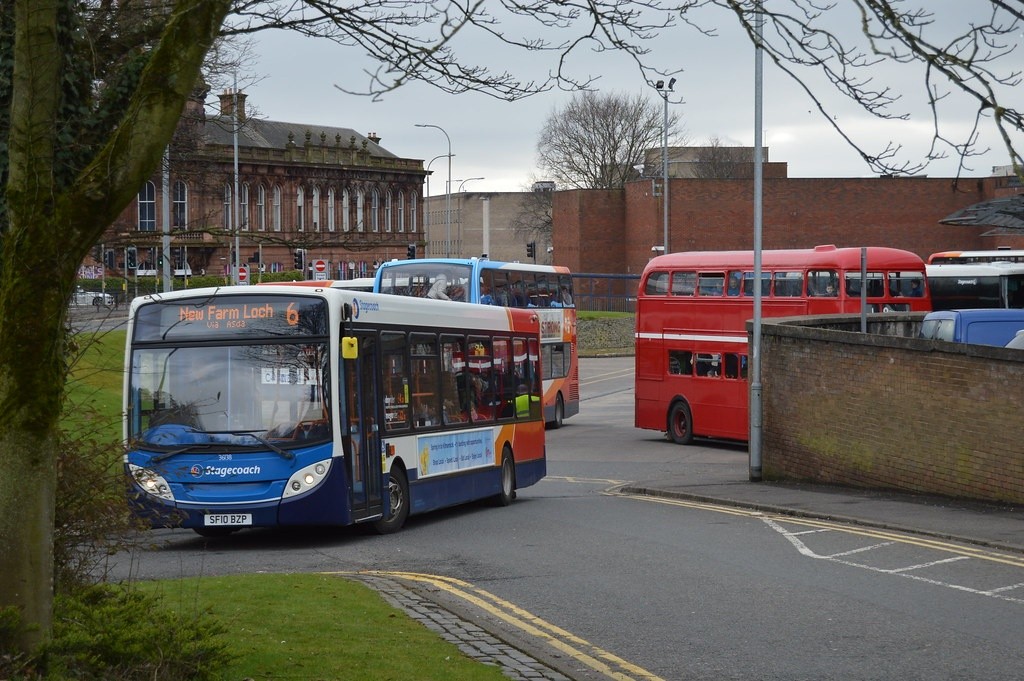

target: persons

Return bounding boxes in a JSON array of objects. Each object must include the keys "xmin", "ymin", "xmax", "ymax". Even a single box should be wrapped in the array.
[
  {"xmin": 386, "ymin": 375, "xmax": 539, "ymax": 430},
  {"xmin": 373, "ymin": 257, "xmax": 389, "ymax": 278},
  {"xmin": 200, "ymin": 266, "xmax": 206, "ymax": 277},
  {"xmin": 667, "ymin": 353, "xmax": 747, "ymax": 380},
  {"xmin": 380, "ymin": 269, "xmax": 573, "ymax": 307},
  {"xmin": 313, "ymin": 227, "xmax": 318, "ymax": 232},
  {"xmin": 672, "ymin": 275, "xmax": 922, "ymax": 299}
]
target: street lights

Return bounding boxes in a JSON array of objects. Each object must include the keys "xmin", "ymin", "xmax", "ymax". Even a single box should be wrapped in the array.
[
  {"xmin": 424, "ymin": 154, "xmax": 456, "ymax": 259},
  {"xmin": 656, "ymin": 77, "xmax": 676, "ymax": 254},
  {"xmin": 415, "ymin": 124, "xmax": 451, "ymax": 259},
  {"xmin": 446, "ymin": 179, "xmax": 463, "ymax": 256},
  {"xmin": 458, "ymin": 177, "xmax": 485, "ymax": 259}
]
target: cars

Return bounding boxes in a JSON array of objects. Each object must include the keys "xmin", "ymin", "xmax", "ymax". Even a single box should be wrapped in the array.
[{"xmin": 68, "ymin": 284, "xmax": 114, "ymax": 307}]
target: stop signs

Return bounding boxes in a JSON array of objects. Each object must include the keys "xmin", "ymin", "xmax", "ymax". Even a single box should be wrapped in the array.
[
  {"xmin": 315, "ymin": 260, "xmax": 326, "ymax": 272},
  {"xmin": 238, "ymin": 267, "xmax": 247, "ymax": 281}
]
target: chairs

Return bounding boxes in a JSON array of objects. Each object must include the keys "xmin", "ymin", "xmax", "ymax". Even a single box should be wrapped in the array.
[
  {"xmin": 670, "ymin": 353, "xmax": 748, "ymax": 379},
  {"xmin": 343, "ymin": 358, "xmax": 537, "ymax": 427},
  {"xmin": 486, "ymin": 288, "xmax": 569, "ymax": 307}
]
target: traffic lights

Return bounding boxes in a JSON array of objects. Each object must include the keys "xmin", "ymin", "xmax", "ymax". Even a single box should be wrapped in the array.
[
  {"xmin": 407, "ymin": 245, "xmax": 416, "ymax": 259},
  {"xmin": 127, "ymin": 247, "xmax": 137, "ymax": 268},
  {"xmin": 147, "ymin": 248, "xmax": 154, "ymax": 264},
  {"xmin": 104, "ymin": 248, "xmax": 114, "ymax": 269},
  {"xmin": 294, "ymin": 249, "xmax": 304, "ymax": 270},
  {"xmin": 527, "ymin": 240, "xmax": 535, "ymax": 260},
  {"xmin": 157, "ymin": 250, "xmax": 163, "ymax": 266}
]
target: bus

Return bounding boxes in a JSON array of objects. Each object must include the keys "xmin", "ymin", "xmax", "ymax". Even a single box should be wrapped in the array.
[
  {"xmin": 635, "ymin": 244, "xmax": 933, "ymax": 446},
  {"xmin": 783, "ymin": 249, "xmax": 1024, "ymax": 350},
  {"xmin": 121, "ymin": 285, "xmax": 547, "ymax": 538},
  {"xmin": 373, "ymin": 256, "xmax": 580, "ymax": 431}
]
